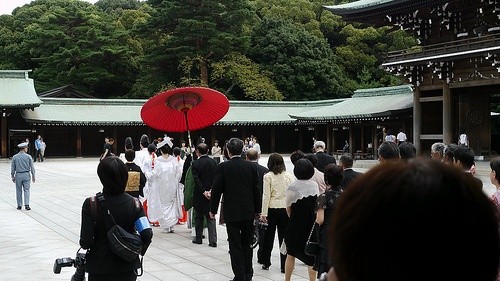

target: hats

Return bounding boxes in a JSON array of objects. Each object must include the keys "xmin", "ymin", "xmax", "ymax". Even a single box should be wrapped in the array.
[
  {"xmin": 314, "ymin": 141, "xmax": 325, "ymax": 149},
  {"xmin": 17, "ymin": 142, "xmax": 28, "ymax": 148}
]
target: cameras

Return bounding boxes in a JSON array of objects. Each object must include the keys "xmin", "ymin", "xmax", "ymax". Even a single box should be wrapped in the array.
[{"xmin": 53, "ymin": 253, "xmax": 89, "ymax": 281}]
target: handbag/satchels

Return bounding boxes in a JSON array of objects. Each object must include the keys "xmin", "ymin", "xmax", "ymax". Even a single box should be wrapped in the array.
[
  {"xmin": 305, "ymin": 221, "xmax": 320, "ymax": 258},
  {"xmin": 107, "ymin": 224, "xmax": 143, "ymax": 264}
]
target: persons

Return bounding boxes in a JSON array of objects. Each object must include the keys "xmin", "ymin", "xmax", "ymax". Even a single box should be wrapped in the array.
[
  {"xmin": 99, "ymin": 127, "xmax": 500, "ymax": 281},
  {"xmin": 25, "ymin": 135, "xmax": 47, "ymax": 162},
  {"xmin": 79, "ymin": 157, "xmax": 153, "ymax": 281},
  {"xmin": 11, "ymin": 143, "xmax": 35, "ymax": 210}
]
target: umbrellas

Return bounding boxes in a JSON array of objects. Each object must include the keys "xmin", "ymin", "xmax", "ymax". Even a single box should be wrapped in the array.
[{"xmin": 140, "ymin": 86, "xmax": 230, "ymax": 161}]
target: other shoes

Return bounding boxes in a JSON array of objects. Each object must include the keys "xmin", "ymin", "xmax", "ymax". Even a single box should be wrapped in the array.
[
  {"xmin": 25, "ymin": 205, "xmax": 29, "ymax": 209},
  {"xmin": 17, "ymin": 206, "xmax": 22, "ymax": 210},
  {"xmin": 209, "ymin": 243, "xmax": 217, "ymax": 247},
  {"xmin": 192, "ymin": 239, "xmax": 199, "ymax": 244},
  {"xmin": 262, "ymin": 263, "xmax": 271, "ymax": 270}
]
